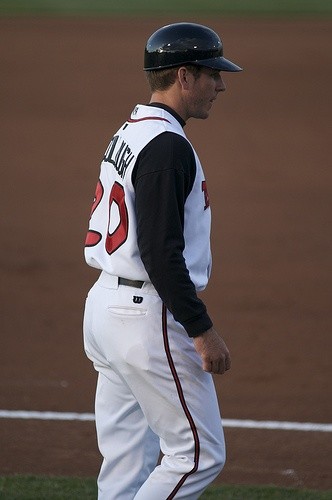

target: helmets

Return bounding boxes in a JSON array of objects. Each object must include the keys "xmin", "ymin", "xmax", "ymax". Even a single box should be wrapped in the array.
[{"xmin": 143, "ymin": 22, "xmax": 243, "ymax": 72}]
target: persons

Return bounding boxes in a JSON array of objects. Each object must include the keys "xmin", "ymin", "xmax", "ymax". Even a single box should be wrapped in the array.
[{"xmin": 83, "ymin": 22, "xmax": 233, "ymax": 500}]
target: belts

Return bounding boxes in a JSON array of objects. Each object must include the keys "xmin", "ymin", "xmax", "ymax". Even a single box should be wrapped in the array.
[{"xmin": 118, "ymin": 277, "xmax": 145, "ymax": 289}]
